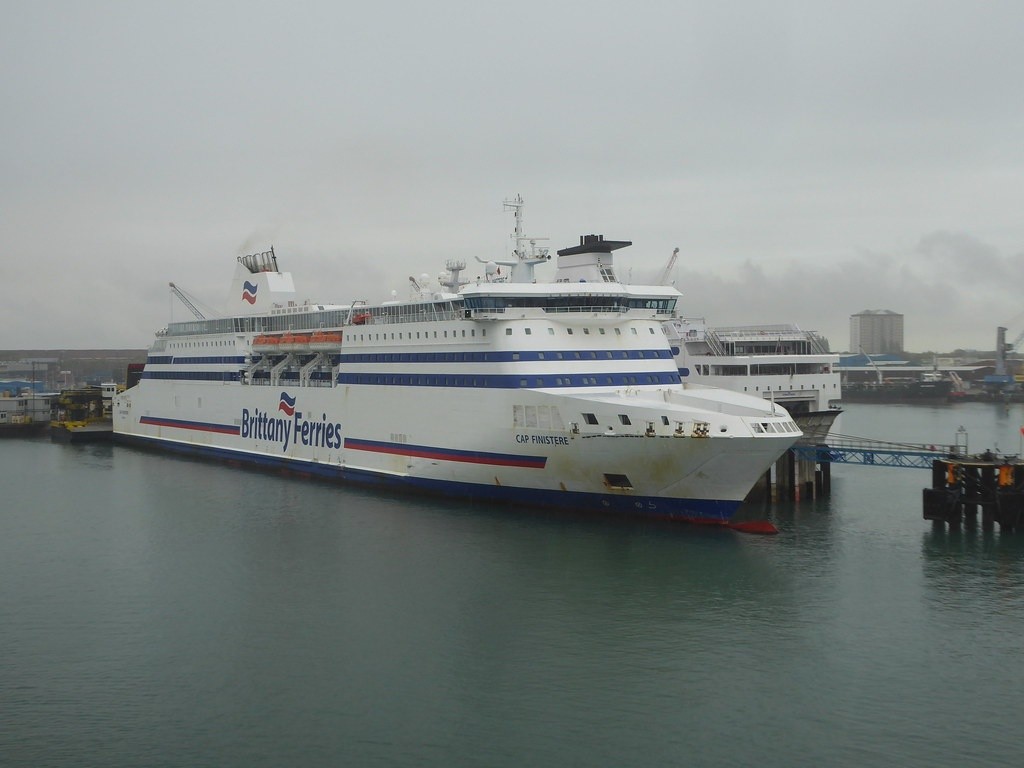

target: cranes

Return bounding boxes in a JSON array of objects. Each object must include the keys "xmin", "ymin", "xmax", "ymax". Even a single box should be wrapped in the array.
[
  {"xmin": 660, "ymin": 248, "xmax": 679, "ymax": 285},
  {"xmin": 170, "ymin": 283, "xmax": 220, "ymax": 319},
  {"xmin": 859, "ymin": 345, "xmax": 883, "ymax": 383}
]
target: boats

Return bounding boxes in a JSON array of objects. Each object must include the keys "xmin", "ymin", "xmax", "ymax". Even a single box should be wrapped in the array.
[
  {"xmin": 114, "ymin": 192, "xmax": 803, "ymax": 527},
  {"xmin": 661, "ymin": 308, "xmax": 844, "ymax": 417}
]
